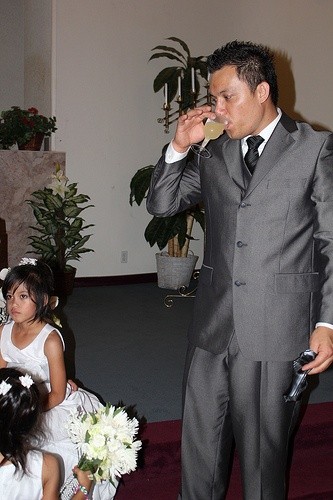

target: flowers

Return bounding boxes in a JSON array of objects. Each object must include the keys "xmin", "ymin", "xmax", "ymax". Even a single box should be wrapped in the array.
[
  {"xmin": 0, "ymin": 106, "xmax": 57, "ymax": 150},
  {"xmin": 18, "ymin": 256, "xmax": 38, "ymax": 267},
  {"xmin": 59, "ymin": 400, "xmax": 142, "ymax": 500},
  {"xmin": 21, "ymin": 159, "xmax": 96, "ymax": 271}
]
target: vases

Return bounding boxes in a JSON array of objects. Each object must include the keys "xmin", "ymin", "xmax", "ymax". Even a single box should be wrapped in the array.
[
  {"xmin": 36, "ymin": 262, "xmax": 77, "ymax": 313},
  {"xmin": 17, "ymin": 131, "xmax": 45, "ymax": 151}
]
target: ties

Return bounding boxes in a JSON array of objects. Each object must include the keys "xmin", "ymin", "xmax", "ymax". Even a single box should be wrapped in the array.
[{"xmin": 244, "ymin": 134, "xmax": 263, "ymax": 176}]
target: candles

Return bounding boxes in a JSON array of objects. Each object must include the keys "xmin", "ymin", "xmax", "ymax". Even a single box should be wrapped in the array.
[
  {"xmin": 191, "ymin": 67, "xmax": 195, "ymax": 92},
  {"xmin": 164, "ymin": 83, "xmax": 168, "ymax": 108},
  {"xmin": 207, "ymin": 65, "xmax": 210, "ymax": 82},
  {"xmin": 177, "ymin": 76, "xmax": 182, "ymax": 101}
]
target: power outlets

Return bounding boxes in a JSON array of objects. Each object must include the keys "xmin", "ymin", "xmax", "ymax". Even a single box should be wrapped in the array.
[{"xmin": 121, "ymin": 250, "xmax": 127, "ymax": 263}]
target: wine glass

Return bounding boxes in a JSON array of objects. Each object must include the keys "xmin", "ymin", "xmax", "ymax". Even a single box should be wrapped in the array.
[{"xmin": 191, "ymin": 106, "xmax": 229, "ymax": 158}]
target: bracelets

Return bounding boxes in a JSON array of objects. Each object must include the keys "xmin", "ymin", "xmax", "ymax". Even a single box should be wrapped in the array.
[{"xmin": 78, "ymin": 486, "xmax": 90, "ymax": 496}]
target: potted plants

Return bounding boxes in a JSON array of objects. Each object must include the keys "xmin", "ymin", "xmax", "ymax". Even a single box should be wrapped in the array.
[{"xmin": 129, "ymin": 35, "xmax": 215, "ymax": 291}]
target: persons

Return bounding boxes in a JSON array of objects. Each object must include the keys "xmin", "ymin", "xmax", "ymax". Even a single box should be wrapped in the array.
[
  {"xmin": 0, "ymin": 367, "xmax": 99, "ymax": 500},
  {"xmin": 147, "ymin": 40, "xmax": 333, "ymax": 500},
  {"xmin": 0, "ymin": 264, "xmax": 78, "ymax": 418}
]
